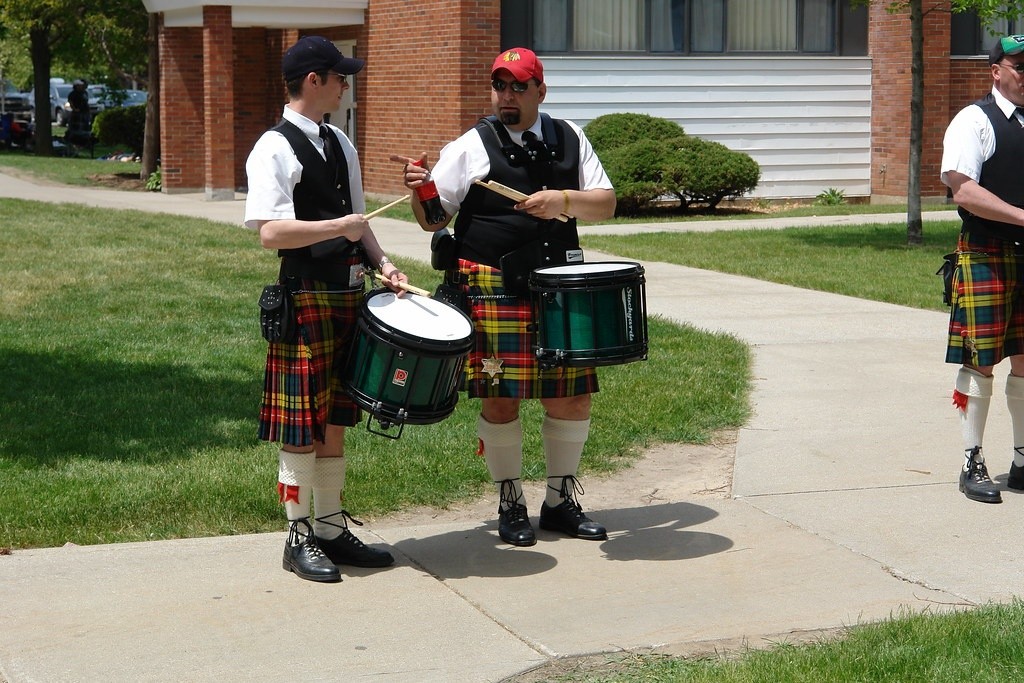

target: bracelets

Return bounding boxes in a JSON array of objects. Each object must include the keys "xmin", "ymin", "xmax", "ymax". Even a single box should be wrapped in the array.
[
  {"xmin": 559, "ymin": 190, "xmax": 569, "ymax": 212},
  {"xmin": 377, "ymin": 258, "xmax": 392, "ymax": 268}
]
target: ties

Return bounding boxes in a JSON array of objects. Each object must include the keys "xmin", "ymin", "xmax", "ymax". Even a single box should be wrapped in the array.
[
  {"xmin": 521, "ymin": 132, "xmax": 544, "ymax": 163},
  {"xmin": 318, "ymin": 127, "xmax": 338, "ymax": 186},
  {"xmin": 1015, "ymin": 107, "xmax": 1024, "ymax": 118}
]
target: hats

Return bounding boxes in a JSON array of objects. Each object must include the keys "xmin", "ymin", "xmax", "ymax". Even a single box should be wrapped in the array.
[
  {"xmin": 491, "ymin": 48, "xmax": 544, "ymax": 83},
  {"xmin": 283, "ymin": 36, "xmax": 364, "ymax": 82},
  {"xmin": 989, "ymin": 35, "xmax": 1024, "ymax": 65}
]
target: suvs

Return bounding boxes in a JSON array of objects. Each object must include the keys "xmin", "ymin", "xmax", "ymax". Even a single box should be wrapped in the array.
[{"xmin": 0, "ymin": 79, "xmax": 35, "ymax": 123}]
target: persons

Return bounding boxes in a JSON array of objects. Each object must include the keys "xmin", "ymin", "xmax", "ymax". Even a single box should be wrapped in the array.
[
  {"xmin": 938, "ymin": 34, "xmax": 1024, "ymax": 504},
  {"xmin": 243, "ymin": 35, "xmax": 410, "ymax": 584},
  {"xmin": 389, "ymin": 48, "xmax": 618, "ymax": 547},
  {"xmin": 6, "ymin": 112, "xmax": 37, "ymax": 139},
  {"xmin": 68, "ymin": 77, "xmax": 93, "ymax": 125}
]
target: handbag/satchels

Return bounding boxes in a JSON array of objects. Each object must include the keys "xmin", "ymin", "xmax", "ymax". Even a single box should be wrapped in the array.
[
  {"xmin": 258, "ymin": 284, "xmax": 297, "ymax": 342},
  {"xmin": 431, "ymin": 228, "xmax": 457, "ymax": 271},
  {"xmin": 941, "ymin": 253, "xmax": 957, "ymax": 304},
  {"xmin": 433, "ymin": 284, "xmax": 468, "ymax": 316}
]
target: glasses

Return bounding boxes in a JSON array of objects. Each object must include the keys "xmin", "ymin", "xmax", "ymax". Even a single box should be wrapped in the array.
[
  {"xmin": 997, "ymin": 62, "xmax": 1024, "ymax": 74},
  {"xmin": 491, "ymin": 79, "xmax": 538, "ymax": 93},
  {"xmin": 316, "ymin": 73, "xmax": 348, "ymax": 83}
]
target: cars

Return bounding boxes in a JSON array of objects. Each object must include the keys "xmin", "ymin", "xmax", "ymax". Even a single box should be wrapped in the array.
[
  {"xmin": 27, "ymin": 79, "xmax": 106, "ymax": 125},
  {"xmin": 86, "ymin": 85, "xmax": 147, "ymax": 110}
]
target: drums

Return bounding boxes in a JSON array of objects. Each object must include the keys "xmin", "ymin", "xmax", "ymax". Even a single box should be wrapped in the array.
[
  {"xmin": 341, "ymin": 286, "xmax": 476, "ymax": 426},
  {"xmin": 532, "ymin": 261, "xmax": 650, "ymax": 368}
]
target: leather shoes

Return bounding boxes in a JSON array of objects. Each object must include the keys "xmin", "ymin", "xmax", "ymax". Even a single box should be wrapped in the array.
[
  {"xmin": 498, "ymin": 507, "xmax": 537, "ymax": 546},
  {"xmin": 959, "ymin": 464, "xmax": 1003, "ymax": 503},
  {"xmin": 283, "ymin": 538, "xmax": 342, "ymax": 582},
  {"xmin": 1008, "ymin": 461, "xmax": 1024, "ymax": 491},
  {"xmin": 314, "ymin": 530, "xmax": 394, "ymax": 567},
  {"xmin": 539, "ymin": 499, "xmax": 606, "ymax": 540}
]
